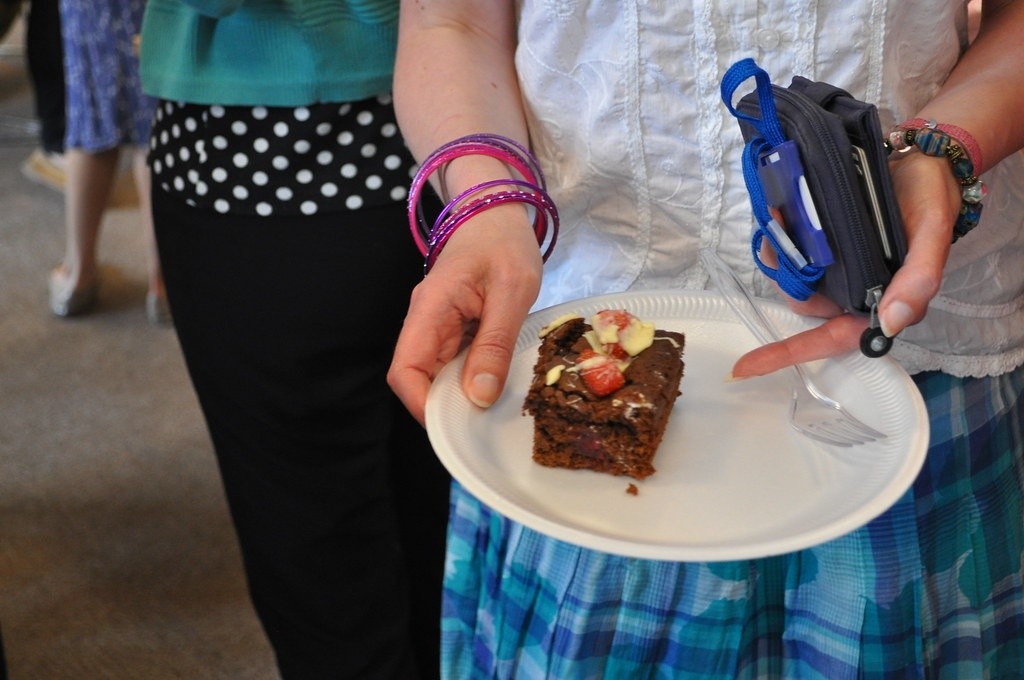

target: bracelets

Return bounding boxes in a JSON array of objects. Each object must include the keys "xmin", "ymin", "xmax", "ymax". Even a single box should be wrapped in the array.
[
  {"xmin": 407, "ymin": 135, "xmax": 561, "ymax": 279},
  {"xmin": 886, "ymin": 120, "xmax": 990, "ymax": 245}
]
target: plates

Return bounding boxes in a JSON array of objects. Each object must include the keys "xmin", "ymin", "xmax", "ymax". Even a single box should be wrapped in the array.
[{"xmin": 425, "ymin": 287, "xmax": 930, "ymax": 561}]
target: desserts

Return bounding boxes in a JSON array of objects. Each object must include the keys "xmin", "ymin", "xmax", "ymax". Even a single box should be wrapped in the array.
[{"xmin": 522, "ymin": 310, "xmax": 686, "ymax": 478}]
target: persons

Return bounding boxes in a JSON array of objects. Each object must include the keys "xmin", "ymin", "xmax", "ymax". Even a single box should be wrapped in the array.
[
  {"xmin": 0, "ymin": 0, "xmax": 165, "ymax": 324},
  {"xmin": 131, "ymin": 0, "xmax": 452, "ymax": 679},
  {"xmin": 385, "ymin": 0, "xmax": 1024, "ymax": 680}
]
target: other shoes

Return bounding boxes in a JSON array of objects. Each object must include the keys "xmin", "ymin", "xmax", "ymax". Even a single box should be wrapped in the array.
[
  {"xmin": 50, "ymin": 261, "xmax": 88, "ymax": 314},
  {"xmin": 144, "ymin": 291, "xmax": 175, "ymax": 327},
  {"xmin": 23, "ymin": 149, "xmax": 141, "ymax": 206}
]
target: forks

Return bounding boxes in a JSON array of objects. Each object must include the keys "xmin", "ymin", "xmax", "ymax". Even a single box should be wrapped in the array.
[{"xmin": 699, "ymin": 247, "xmax": 888, "ymax": 448}]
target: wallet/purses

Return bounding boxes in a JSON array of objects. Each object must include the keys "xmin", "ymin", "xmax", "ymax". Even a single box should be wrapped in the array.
[{"xmin": 735, "ymin": 76, "xmax": 908, "ymax": 356}]
